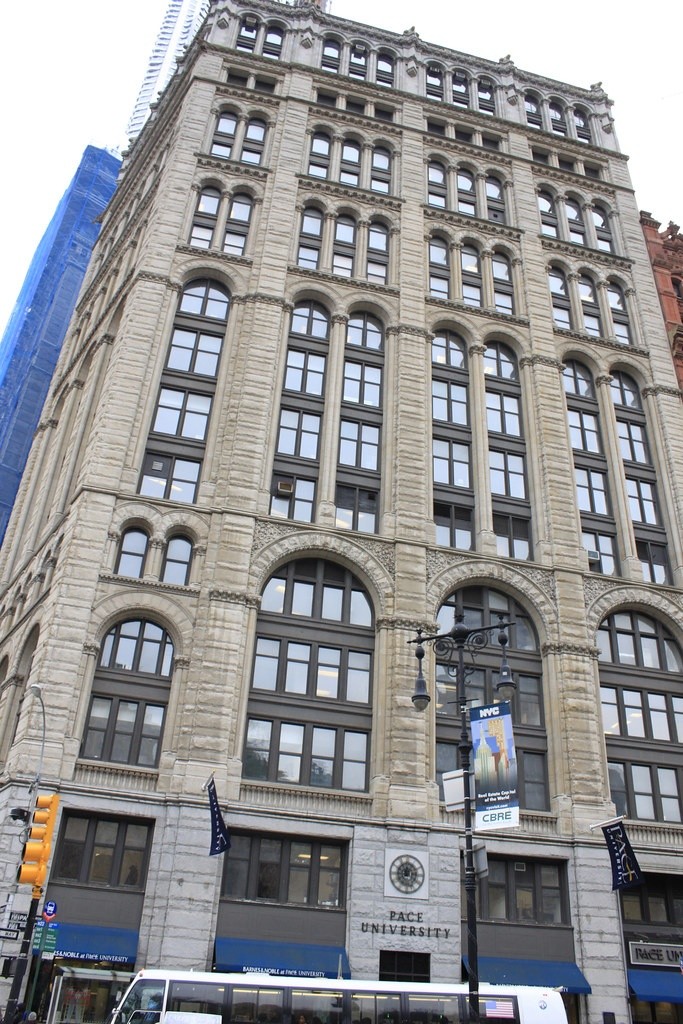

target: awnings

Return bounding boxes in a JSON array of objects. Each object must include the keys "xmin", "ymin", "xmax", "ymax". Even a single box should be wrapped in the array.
[
  {"xmin": 461, "ymin": 954, "xmax": 592, "ymax": 994},
  {"xmin": 212, "ymin": 934, "xmax": 352, "ymax": 984},
  {"xmin": 32, "ymin": 920, "xmax": 140, "ymax": 964},
  {"xmin": 626, "ymin": 968, "xmax": 683, "ymax": 1003}
]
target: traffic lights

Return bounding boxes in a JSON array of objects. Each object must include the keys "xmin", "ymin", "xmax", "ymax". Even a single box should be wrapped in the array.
[{"xmin": 16, "ymin": 793, "xmax": 61, "ymax": 887}]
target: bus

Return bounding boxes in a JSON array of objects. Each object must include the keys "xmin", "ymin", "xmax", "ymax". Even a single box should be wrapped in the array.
[{"xmin": 110, "ymin": 969, "xmax": 568, "ymax": 1024}]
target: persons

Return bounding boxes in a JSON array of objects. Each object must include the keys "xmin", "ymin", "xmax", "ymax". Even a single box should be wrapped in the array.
[
  {"xmin": 132, "ymin": 992, "xmax": 163, "ymax": 1024},
  {"xmin": 296, "ymin": 1014, "xmax": 309, "ymax": 1024},
  {"xmin": 260, "ymin": 1008, "xmax": 280, "ymax": 1024}
]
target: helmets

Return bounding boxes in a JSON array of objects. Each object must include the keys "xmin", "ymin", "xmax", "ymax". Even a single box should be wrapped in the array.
[{"xmin": 28, "ymin": 1012, "xmax": 36, "ymax": 1020}]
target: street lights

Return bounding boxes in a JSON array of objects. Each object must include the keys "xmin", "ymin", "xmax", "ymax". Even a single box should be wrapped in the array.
[
  {"xmin": 24, "ymin": 684, "xmax": 46, "ymax": 829},
  {"xmin": 407, "ymin": 612, "xmax": 518, "ymax": 1021}
]
